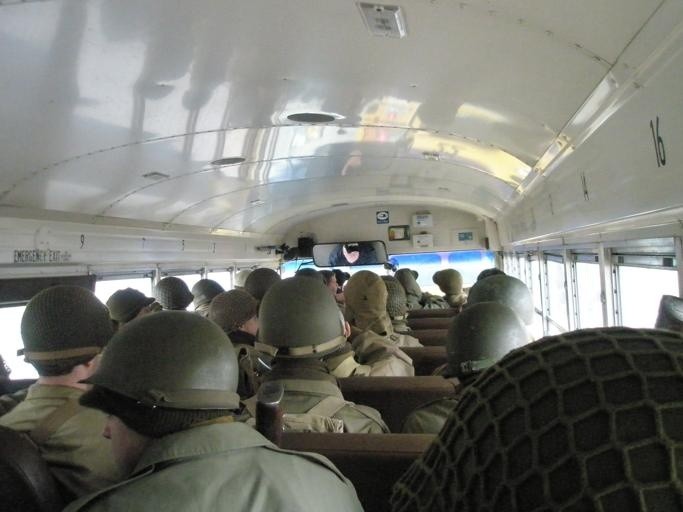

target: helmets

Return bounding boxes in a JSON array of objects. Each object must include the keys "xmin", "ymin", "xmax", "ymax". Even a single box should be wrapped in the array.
[
  {"xmin": 244, "ymin": 268, "xmax": 280, "ymax": 300},
  {"xmin": 20, "ymin": 286, "xmax": 111, "ymax": 369},
  {"xmin": 468, "ymin": 275, "xmax": 533, "ymax": 324},
  {"xmin": 191, "ymin": 279, "xmax": 225, "ymax": 310},
  {"xmin": 382, "ymin": 275, "xmax": 408, "ymax": 317},
  {"xmin": 106, "ymin": 289, "xmax": 155, "ymax": 321},
  {"xmin": 395, "ymin": 268, "xmax": 419, "ymax": 290},
  {"xmin": 433, "ymin": 270, "xmax": 462, "ymax": 296},
  {"xmin": 382, "ymin": 321, "xmax": 683, "ymax": 512},
  {"xmin": 254, "ymin": 275, "xmax": 348, "ymax": 358},
  {"xmin": 207, "ymin": 289, "xmax": 258, "ymax": 334},
  {"xmin": 79, "ymin": 310, "xmax": 242, "ymax": 433},
  {"xmin": 441, "ymin": 301, "xmax": 527, "ymax": 379},
  {"xmin": 154, "ymin": 277, "xmax": 193, "ymax": 310}
]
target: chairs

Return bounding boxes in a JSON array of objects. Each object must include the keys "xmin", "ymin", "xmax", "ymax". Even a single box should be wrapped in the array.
[{"xmin": 13, "ymin": 305, "xmax": 464, "ymax": 512}]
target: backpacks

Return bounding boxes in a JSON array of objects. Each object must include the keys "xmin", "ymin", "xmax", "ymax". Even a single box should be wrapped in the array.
[{"xmin": 243, "ymin": 390, "xmax": 350, "ymax": 437}]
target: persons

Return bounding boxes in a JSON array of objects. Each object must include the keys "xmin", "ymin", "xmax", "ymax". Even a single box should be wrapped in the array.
[{"xmin": 328, "ymin": 242, "xmax": 377, "ymax": 266}]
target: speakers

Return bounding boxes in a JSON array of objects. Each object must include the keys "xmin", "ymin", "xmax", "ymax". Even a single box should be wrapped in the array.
[{"xmin": 297, "ymin": 237, "xmax": 313, "ymax": 257}]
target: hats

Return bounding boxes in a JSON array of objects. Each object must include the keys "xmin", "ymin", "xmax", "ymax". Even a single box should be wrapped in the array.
[
  {"xmin": 344, "ymin": 271, "xmax": 389, "ymax": 319},
  {"xmin": 343, "ymin": 242, "xmax": 361, "ymax": 254},
  {"xmin": 234, "ymin": 269, "xmax": 252, "ymax": 287}
]
